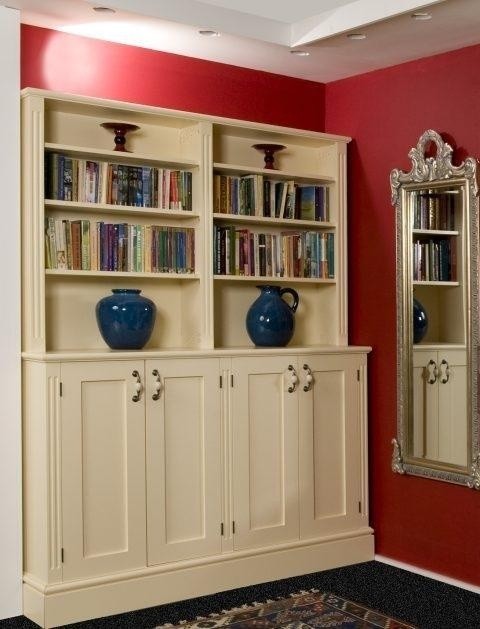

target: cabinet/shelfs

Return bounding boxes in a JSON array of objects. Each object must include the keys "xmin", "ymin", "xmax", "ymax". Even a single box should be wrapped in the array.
[
  {"xmin": 409, "ymin": 184, "xmax": 469, "ymax": 467},
  {"xmin": 23, "ymin": 352, "xmax": 223, "ymax": 627},
  {"xmin": 19, "ymin": 85, "xmax": 208, "ymax": 351},
  {"xmin": 224, "ymin": 349, "xmax": 377, "ymax": 608},
  {"xmin": 209, "ymin": 115, "xmax": 351, "ymax": 348}
]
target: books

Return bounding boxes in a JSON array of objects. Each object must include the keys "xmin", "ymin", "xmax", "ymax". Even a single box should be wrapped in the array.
[
  {"xmin": 40, "ymin": 150, "xmax": 336, "ymax": 281},
  {"xmin": 410, "ymin": 189, "xmax": 459, "ymax": 281}
]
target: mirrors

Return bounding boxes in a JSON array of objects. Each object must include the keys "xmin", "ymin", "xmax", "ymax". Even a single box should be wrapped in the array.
[{"xmin": 389, "ymin": 128, "xmax": 480, "ymax": 491}]
